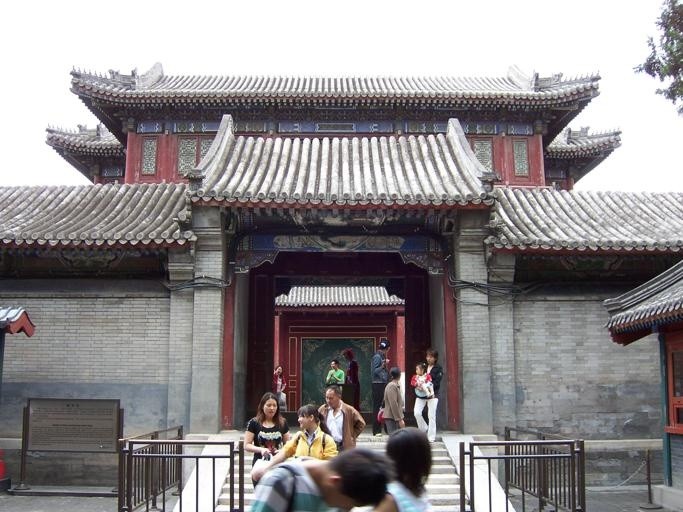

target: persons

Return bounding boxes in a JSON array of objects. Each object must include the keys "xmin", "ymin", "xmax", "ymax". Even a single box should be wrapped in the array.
[{"xmin": 243, "ymin": 339, "xmax": 444, "ymax": 512}]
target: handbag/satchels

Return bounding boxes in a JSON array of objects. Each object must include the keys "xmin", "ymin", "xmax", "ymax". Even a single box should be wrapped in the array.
[
  {"xmin": 376, "ymin": 408, "xmax": 384, "ymax": 424},
  {"xmin": 250, "ymin": 454, "xmax": 272, "ymax": 475}
]
